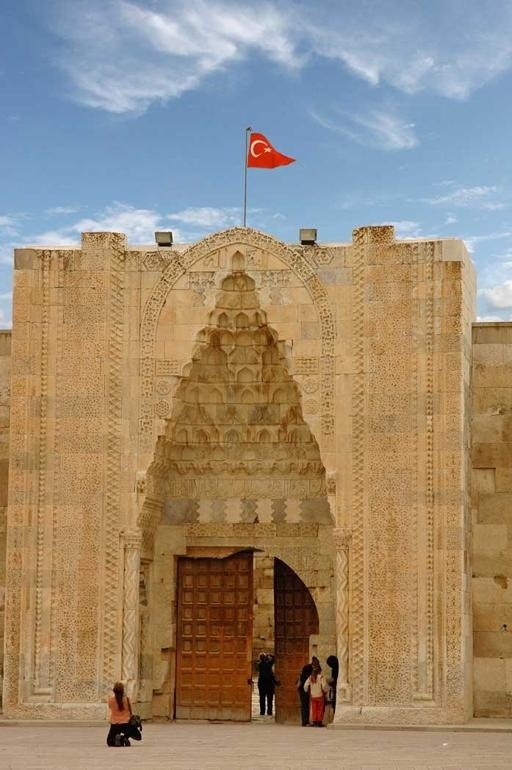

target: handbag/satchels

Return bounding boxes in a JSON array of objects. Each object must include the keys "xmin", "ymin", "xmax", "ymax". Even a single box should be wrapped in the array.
[{"xmin": 130, "ymin": 715, "xmax": 141, "ymax": 729}]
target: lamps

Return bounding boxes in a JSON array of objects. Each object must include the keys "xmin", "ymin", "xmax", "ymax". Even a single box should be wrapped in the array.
[
  {"xmin": 154, "ymin": 231, "xmax": 173, "ymax": 247},
  {"xmin": 299, "ymin": 228, "xmax": 317, "ymax": 245}
]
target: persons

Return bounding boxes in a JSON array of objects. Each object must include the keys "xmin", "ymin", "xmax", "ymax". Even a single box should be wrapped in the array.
[
  {"xmin": 297, "ymin": 657, "xmax": 319, "ymax": 727},
  {"xmin": 304, "ymin": 666, "xmax": 328, "ymax": 726},
  {"xmin": 326, "ymin": 654, "xmax": 339, "ymax": 714},
  {"xmin": 256, "ymin": 652, "xmax": 276, "ymax": 719},
  {"xmin": 106, "ymin": 682, "xmax": 135, "ymax": 747}
]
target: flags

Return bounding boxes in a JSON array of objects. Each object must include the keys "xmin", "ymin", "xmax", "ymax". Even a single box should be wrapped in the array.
[{"xmin": 247, "ymin": 133, "xmax": 296, "ymax": 169}]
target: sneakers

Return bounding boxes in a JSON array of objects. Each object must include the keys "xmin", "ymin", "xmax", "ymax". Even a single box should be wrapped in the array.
[
  {"xmin": 115, "ymin": 734, "xmax": 130, "ymax": 746},
  {"xmin": 303, "ymin": 723, "xmax": 325, "ymax": 727}
]
